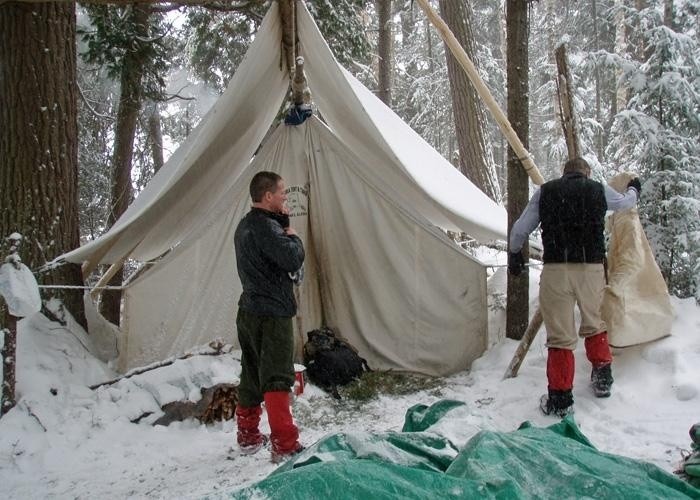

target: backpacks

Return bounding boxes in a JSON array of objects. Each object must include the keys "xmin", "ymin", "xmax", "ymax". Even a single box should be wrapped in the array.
[{"xmin": 303, "ymin": 328, "xmax": 363, "ymax": 388}]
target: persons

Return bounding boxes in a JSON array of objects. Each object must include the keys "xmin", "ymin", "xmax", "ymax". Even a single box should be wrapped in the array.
[
  {"xmin": 507, "ymin": 157, "xmax": 642, "ymax": 420},
  {"xmin": 233, "ymin": 171, "xmax": 306, "ymax": 462}
]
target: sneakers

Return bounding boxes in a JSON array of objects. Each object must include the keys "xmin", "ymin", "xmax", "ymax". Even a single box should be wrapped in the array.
[
  {"xmin": 271, "ymin": 447, "xmax": 305, "ymax": 463},
  {"xmin": 240, "ymin": 433, "xmax": 270, "ymax": 455}
]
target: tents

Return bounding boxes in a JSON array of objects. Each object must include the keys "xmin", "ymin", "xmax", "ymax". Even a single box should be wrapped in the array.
[{"xmin": 62, "ymin": 1, "xmax": 540, "ymax": 377}]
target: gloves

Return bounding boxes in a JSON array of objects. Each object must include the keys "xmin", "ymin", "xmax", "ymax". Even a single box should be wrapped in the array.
[{"xmin": 628, "ymin": 178, "xmax": 641, "ymax": 191}]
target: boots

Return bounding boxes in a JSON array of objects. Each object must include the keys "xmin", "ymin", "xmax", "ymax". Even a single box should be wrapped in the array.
[
  {"xmin": 591, "ymin": 364, "xmax": 613, "ymax": 397},
  {"xmin": 541, "ymin": 387, "xmax": 574, "ymax": 417}
]
